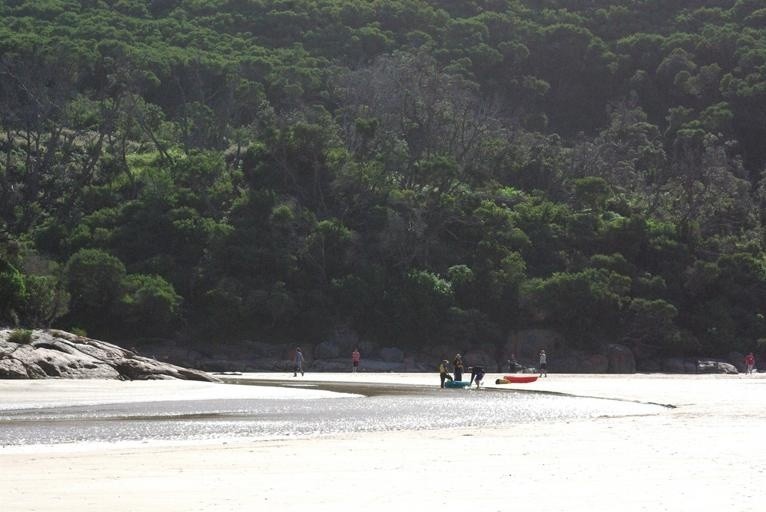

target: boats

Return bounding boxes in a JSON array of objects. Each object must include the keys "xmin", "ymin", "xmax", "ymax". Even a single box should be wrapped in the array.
[
  {"xmin": 446, "ymin": 380, "xmax": 471, "ymax": 390},
  {"xmin": 503, "ymin": 375, "xmax": 538, "ymax": 383}
]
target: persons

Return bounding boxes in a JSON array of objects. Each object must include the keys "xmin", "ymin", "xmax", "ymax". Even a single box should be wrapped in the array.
[
  {"xmin": 453, "ymin": 353, "xmax": 465, "ymax": 381},
  {"xmin": 352, "ymin": 348, "xmax": 360, "ymax": 373},
  {"xmin": 440, "ymin": 359, "xmax": 453, "ymax": 388},
  {"xmin": 539, "ymin": 350, "xmax": 547, "ymax": 377},
  {"xmin": 293, "ymin": 347, "xmax": 304, "ymax": 377},
  {"xmin": 468, "ymin": 366, "xmax": 485, "ymax": 389},
  {"xmin": 746, "ymin": 352, "xmax": 755, "ymax": 374}
]
